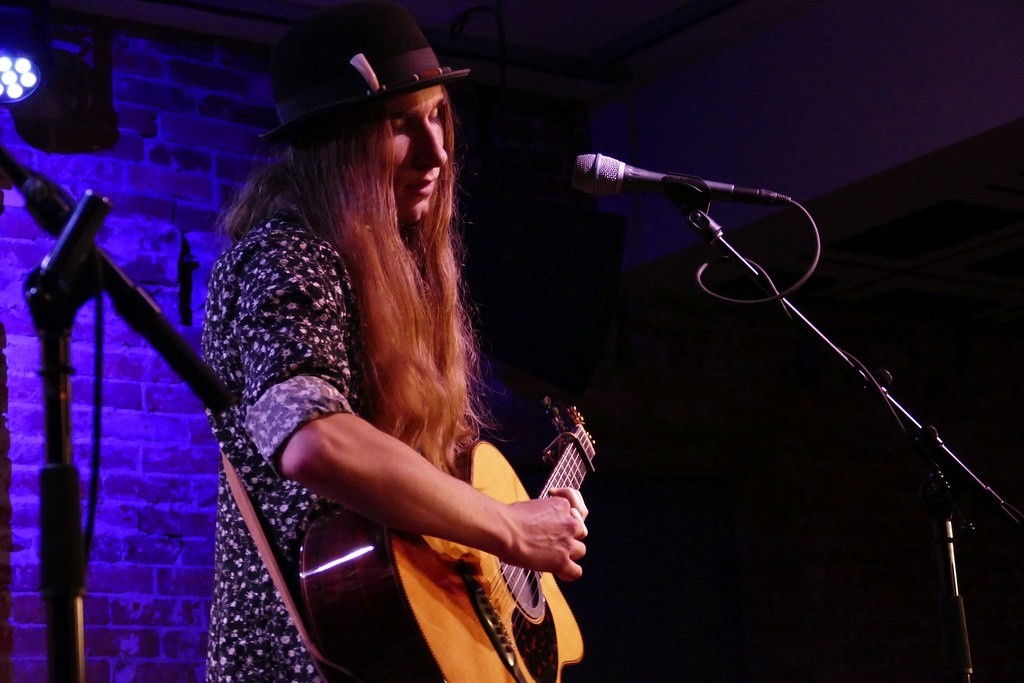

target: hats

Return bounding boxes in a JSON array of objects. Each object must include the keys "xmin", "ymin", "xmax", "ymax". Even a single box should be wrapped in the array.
[{"xmin": 258, "ymin": 0, "xmax": 485, "ymax": 144}]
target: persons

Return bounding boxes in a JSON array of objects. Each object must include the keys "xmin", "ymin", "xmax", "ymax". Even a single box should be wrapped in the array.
[{"xmin": 200, "ymin": 2, "xmax": 591, "ymax": 683}]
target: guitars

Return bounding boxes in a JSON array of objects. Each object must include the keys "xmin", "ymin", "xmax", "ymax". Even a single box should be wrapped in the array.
[{"xmin": 300, "ymin": 396, "xmax": 596, "ymax": 683}]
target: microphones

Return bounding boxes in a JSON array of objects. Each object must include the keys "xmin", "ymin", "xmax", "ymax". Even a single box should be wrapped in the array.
[{"xmin": 571, "ymin": 153, "xmax": 792, "ymax": 209}]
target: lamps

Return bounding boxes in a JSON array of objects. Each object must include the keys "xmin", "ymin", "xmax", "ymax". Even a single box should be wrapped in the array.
[{"xmin": 0, "ymin": 10, "xmax": 42, "ymax": 107}]
